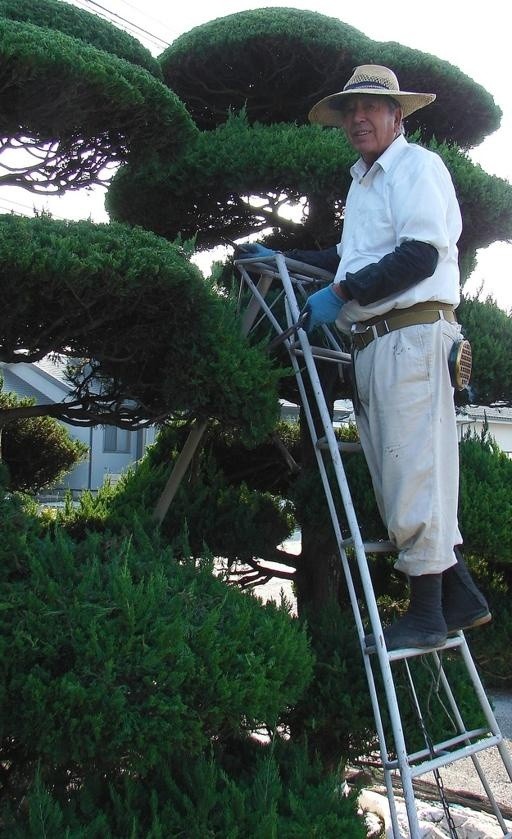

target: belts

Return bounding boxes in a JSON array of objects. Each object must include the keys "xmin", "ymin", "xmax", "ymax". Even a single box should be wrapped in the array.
[{"xmin": 351, "ymin": 310, "xmax": 457, "ymax": 350}]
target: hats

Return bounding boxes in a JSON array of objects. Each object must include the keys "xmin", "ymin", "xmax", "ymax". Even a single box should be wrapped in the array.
[{"xmin": 308, "ymin": 65, "xmax": 437, "ymax": 126}]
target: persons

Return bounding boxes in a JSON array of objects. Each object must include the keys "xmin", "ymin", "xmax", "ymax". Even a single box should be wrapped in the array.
[{"xmin": 232, "ymin": 62, "xmax": 495, "ymax": 655}]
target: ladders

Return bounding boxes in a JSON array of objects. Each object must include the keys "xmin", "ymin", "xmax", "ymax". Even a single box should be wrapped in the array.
[{"xmin": 229, "ymin": 254, "xmax": 510, "ymax": 839}]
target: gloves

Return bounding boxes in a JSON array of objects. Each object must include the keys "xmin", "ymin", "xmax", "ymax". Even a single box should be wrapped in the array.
[
  {"xmin": 233, "ymin": 244, "xmax": 278, "ymax": 269},
  {"xmin": 301, "ymin": 283, "xmax": 345, "ymax": 332}
]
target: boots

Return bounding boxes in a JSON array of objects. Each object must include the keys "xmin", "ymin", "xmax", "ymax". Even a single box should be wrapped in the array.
[
  {"xmin": 442, "ymin": 546, "xmax": 493, "ymax": 637},
  {"xmin": 365, "ymin": 574, "xmax": 447, "ymax": 650}
]
